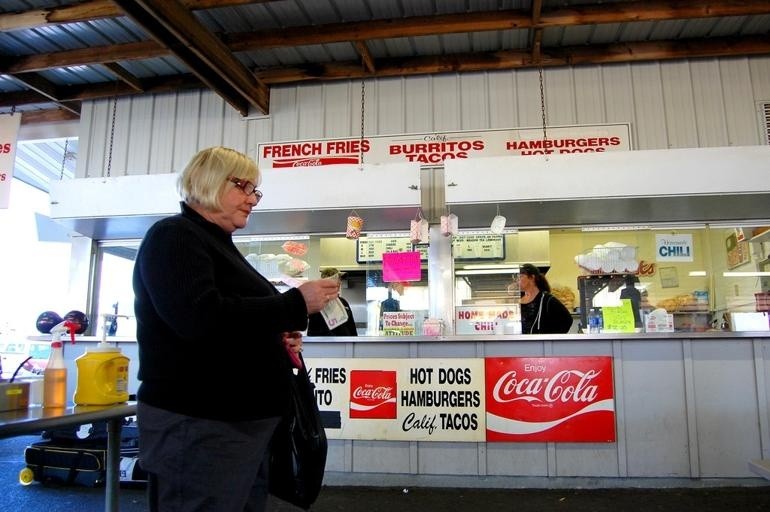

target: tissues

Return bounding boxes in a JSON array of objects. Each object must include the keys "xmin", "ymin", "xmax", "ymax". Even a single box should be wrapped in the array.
[{"xmin": 644, "ymin": 309, "xmax": 675, "ymax": 331}]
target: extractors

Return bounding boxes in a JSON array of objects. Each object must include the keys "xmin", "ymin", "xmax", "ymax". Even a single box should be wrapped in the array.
[{"xmin": 318, "ymin": 230, "xmax": 551, "ymax": 276}]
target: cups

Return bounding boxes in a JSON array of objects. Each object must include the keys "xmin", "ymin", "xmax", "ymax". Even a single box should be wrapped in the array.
[{"xmin": 576, "ymin": 238, "xmax": 642, "ymax": 273}]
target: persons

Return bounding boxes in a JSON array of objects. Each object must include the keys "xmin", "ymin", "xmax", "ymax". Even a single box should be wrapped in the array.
[
  {"xmin": 517, "ymin": 264, "xmax": 573, "ymax": 333},
  {"xmin": 132, "ymin": 147, "xmax": 340, "ymax": 511},
  {"xmin": 307, "ymin": 267, "xmax": 358, "ymax": 337}
]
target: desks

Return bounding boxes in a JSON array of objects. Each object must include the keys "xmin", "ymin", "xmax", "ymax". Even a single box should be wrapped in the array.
[{"xmin": 1, "ymin": 401, "xmax": 158, "ymax": 511}]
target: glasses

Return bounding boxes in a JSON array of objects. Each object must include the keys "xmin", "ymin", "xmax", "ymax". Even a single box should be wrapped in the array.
[{"xmin": 226, "ymin": 175, "xmax": 263, "ymax": 202}]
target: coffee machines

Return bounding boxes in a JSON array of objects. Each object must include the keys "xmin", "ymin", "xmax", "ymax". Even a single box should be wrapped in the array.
[{"xmin": 577, "ymin": 270, "xmax": 646, "ymax": 333}]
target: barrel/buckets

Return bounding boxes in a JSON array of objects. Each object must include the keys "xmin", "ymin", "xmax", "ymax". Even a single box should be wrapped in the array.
[{"xmin": 74, "ymin": 341, "xmax": 130, "ymax": 405}]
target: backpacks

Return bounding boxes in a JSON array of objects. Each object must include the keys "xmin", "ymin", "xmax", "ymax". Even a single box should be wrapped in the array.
[{"xmin": 267, "ymin": 351, "xmax": 328, "ymax": 512}]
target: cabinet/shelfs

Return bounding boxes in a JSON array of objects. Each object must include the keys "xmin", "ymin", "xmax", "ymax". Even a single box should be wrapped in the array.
[{"xmin": 577, "ymin": 272, "xmax": 638, "ymax": 326}]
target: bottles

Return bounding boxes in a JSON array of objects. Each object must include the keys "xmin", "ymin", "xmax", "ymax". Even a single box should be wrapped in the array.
[
  {"xmin": 597, "ymin": 308, "xmax": 605, "ymax": 329},
  {"xmin": 587, "ymin": 309, "xmax": 598, "ymax": 334}
]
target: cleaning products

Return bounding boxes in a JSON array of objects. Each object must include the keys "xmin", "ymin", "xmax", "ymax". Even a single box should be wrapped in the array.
[{"xmin": 44, "ymin": 320, "xmax": 81, "ymax": 409}]
